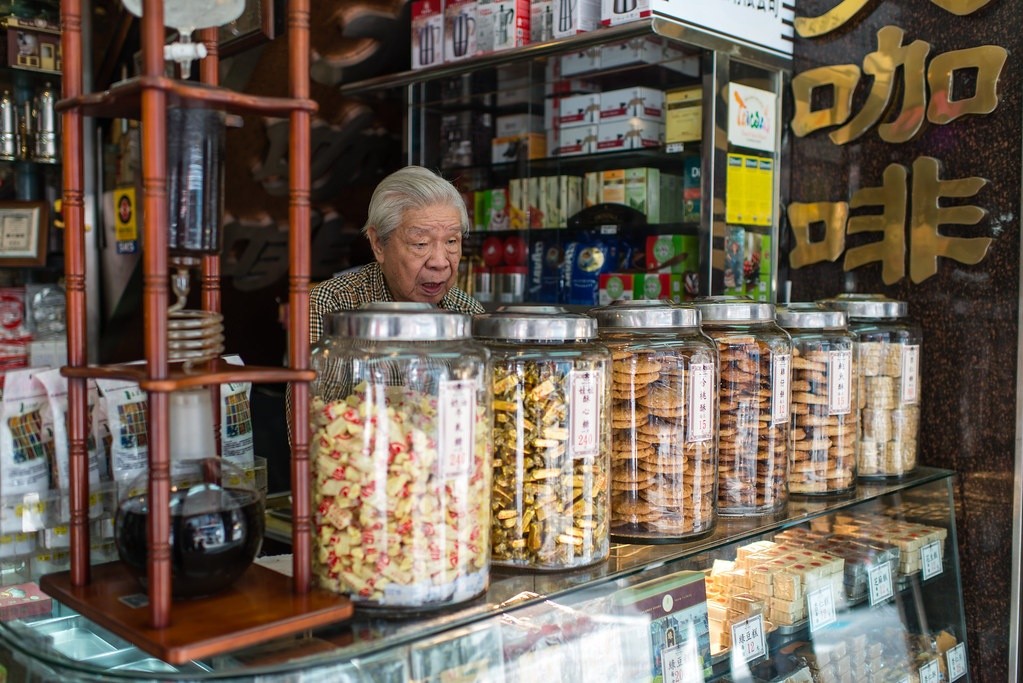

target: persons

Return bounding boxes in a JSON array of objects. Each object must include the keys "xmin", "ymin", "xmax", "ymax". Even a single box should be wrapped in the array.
[{"xmin": 287, "ymin": 167, "xmax": 486, "ymax": 447}]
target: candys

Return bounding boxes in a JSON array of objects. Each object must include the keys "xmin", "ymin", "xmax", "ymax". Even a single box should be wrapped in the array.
[{"xmin": 313, "ymin": 357, "xmax": 609, "ymax": 605}]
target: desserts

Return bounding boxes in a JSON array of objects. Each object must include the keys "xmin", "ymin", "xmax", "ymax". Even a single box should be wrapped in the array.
[{"xmin": 706, "ymin": 510, "xmax": 956, "ymax": 682}]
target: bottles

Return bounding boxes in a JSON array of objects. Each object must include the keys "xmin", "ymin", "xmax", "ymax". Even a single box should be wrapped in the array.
[{"xmin": 299, "ymin": 291, "xmax": 924, "ymax": 619}]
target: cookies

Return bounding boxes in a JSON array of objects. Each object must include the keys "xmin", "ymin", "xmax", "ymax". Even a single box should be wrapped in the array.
[{"xmin": 610, "ymin": 339, "xmax": 921, "ymax": 533}]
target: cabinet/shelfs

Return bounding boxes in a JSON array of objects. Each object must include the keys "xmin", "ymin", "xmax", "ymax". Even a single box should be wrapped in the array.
[
  {"xmin": 337, "ymin": 14, "xmax": 796, "ymax": 311},
  {"xmin": 0, "ymin": 0, "xmax": 355, "ymax": 664},
  {"xmin": 0, "ymin": 466, "xmax": 974, "ymax": 683}
]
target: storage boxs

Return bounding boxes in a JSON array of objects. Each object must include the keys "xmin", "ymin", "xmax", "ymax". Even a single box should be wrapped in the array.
[
  {"xmin": 0, "ymin": 582, "xmax": 51, "ymax": 621},
  {"xmin": 489, "ymin": 38, "xmax": 728, "ymax": 164},
  {"xmin": 468, "ymin": 167, "xmax": 709, "ymax": 307},
  {"xmin": 410, "ymin": 0, "xmax": 797, "ymax": 70}
]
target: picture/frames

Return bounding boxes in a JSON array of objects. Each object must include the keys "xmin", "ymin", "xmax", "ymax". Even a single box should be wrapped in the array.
[{"xmin": 0, "ymin": 201, "xmax": 49, "ymax": 267}]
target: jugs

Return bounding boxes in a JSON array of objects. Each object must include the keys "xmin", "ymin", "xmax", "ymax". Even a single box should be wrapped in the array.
[
  {"xmin": 451, "ymin": 11, "xmax": 476, "ymax": 57},
  {"xmin": 493, "ymin": 4, "xmax": 514, "ymax": 45},
  {"xmin": 559, "ymin": 0, "xmax": 574, "ymax": 32},
  {"xmin": 540, "ymin": 5, "xmax": 553, "ymax": 42},
  {"xmin": 419, "ymin": 22, "xmax": 441, "ymax": 65}
]
